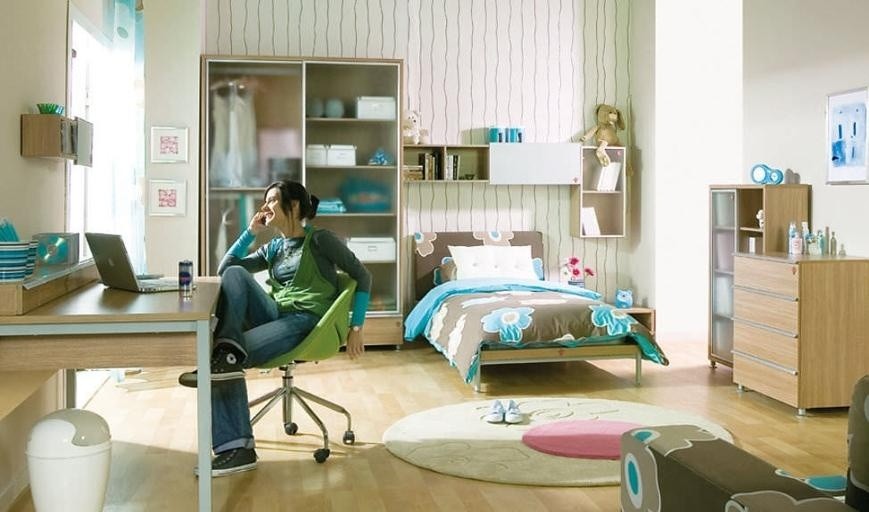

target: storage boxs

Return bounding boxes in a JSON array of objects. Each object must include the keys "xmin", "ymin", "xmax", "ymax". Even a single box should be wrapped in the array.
[
  {"xmin": 304, "ymin": 144, "xmax": 359, "ymax": 170},
  {"xmin": 30, "ymin": 233, "xmax": 79, "ymax": 268},
  {"xmin": 343, "ymin": 234, "xmax": 398, "ymax": 265},
  {"xmin": 353, "ymin": 93, "xmax": 397, "ymax": 122}
]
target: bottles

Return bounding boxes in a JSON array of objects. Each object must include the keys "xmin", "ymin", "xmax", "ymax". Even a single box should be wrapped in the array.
[
  {"xmin": 825, "ymin": 226, "xmax": 846, "ymax": 255},
  {"xmin": 177, "ymin": 260, "xmax": 194, "ymax": 298},
  {"xmin": 789, "ymin": 220, "xmax": 826, "ymax": 257}
]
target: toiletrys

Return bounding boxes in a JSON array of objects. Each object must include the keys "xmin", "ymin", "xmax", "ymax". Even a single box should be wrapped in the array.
[
  {"xmin": 788, "ymin": 221, "xmax": 846, "ymax": 256},
  {"xmin": 792, "ymin": 232, "xmax": 803, "ymax": 254}
]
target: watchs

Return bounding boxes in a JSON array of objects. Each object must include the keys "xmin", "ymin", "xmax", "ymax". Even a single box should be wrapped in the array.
[{"xmin": 350, "ymin": 325, "xmax": 360, "ymax": 334}]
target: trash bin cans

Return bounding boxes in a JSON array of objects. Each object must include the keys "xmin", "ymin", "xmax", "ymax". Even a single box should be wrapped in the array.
[{"xmin": 24, "ymin": 408, "xmax": 113, "ymax": 512}]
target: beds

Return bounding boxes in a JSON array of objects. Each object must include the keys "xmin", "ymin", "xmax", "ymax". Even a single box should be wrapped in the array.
[{"xmin": 411, "ymin": 228, "xmax": 645, "ymax": 395}]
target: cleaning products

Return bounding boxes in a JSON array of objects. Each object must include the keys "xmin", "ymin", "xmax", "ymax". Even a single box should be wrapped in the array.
[
  {"xmin": 801, "ymin": 222, "xmax": 809, "ymax": 252},
  {"xmin": 817, "ymin": 230, "xmax": 824, "ymax": 254},
  {"xmin": 788, "ymin": 223, "xmax": 796, "ymax": 253}
]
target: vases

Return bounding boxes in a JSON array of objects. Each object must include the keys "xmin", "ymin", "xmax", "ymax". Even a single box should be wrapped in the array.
[{"xmin": 568, "ymin": 277, "xmax": 586, "ymax": 287}]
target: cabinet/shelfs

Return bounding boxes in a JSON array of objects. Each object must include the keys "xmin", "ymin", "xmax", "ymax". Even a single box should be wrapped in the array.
[
  {"xmin": 705, "ymin": 182, "xmax": 815, "ymax": 376},
  {"xmin": 402, "ymin": 142, "xmax": 491, "ymax": 184},
  {"xmin": 21, "ymin": 111, "xmax": 79, "ymax": 161},
  {"xmin": 488, "ymin": 139, "xmax": 582, "ymax": 188},
  {"xmin": 729, "ymin": 246, "xmax": 868, "ymax": 420},
  {"xmin": 568, "ymin": 142, "xmax": 628, "ymax": 239},
  {"xmin": 199, "ymin": 52, "xmax": 407, "ymax": 353}
]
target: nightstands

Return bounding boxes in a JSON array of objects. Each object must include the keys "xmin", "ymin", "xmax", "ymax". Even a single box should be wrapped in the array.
[{"xmin": 607, "ymin": 304, "xmax": 656, "ymax": 346}]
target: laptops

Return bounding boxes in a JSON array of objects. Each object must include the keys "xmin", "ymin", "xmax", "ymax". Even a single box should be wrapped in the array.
[{"xmin": 84, "ymin": 231, "xmax": 197, "ymax": 293}]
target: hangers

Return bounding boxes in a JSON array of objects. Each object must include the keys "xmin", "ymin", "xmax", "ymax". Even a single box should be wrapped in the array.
[
  {"xmin": 222, "ymin": 199, "xmax": 241, "ymax": 227},
  {"xmin": 209, "ymin": 74, "xmax": 269, "ymax": 94}
]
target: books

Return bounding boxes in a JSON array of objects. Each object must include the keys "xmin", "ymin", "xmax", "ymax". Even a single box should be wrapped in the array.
[
  {"xmin": 581, "ymin": 161, "xmax": 622, "ymax": 236},
  {"xmin": 402, "ymin": 146, "xmax": 462, "ymax": 181}
]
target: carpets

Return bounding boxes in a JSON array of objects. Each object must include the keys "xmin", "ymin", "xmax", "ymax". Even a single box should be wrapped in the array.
[{"xmin": 381, "ymin": 393, "xmax": 740, "ymax": 492}]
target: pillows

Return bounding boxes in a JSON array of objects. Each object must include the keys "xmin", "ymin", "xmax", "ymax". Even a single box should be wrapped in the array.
[
  {"xmin": 447, "ymin": 242, "xmax": 543, "ymax": 286},
  {"xmin": 438, "ymin": 261, "xmax": 459, "ymax": 282}
]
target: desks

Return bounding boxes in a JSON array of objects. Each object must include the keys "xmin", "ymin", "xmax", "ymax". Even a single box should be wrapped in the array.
[{"xmin": 1, "ymin": 271, "xmax": 224, "ymax": 512}]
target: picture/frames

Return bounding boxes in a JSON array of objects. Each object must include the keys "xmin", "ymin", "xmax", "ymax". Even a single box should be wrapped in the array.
[
  {"xmin": 150, "ymin": 125, "xmax": 189, "ymax": 165},
  {"xmin": 147, "ymin": 179, "xmax": 186, "ymax": 216},
  {"xmin": 823, "ymin": 86, "xmax": 869, "ymax": 184}
]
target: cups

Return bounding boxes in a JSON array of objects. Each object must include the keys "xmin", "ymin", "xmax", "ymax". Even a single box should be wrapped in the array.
[{"xmin": 489, "ymin": 126, "xmax": 526, "ymax": 143}]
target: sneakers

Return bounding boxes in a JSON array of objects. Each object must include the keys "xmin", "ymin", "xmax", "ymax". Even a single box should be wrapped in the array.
[
  {"xmin": 505, "ymin": 399, "xmax": 522, "ymax": 424},
  {"xmin": 486, "ymin": 400, "xmax": 505, "ymax": 423},
  {"xmin": 178, "ymin": 345, "xmax": 246, "ymax": 388},
  {"xmin": 193, "ymin": 448, "xmax": 258, "ymax": 477}
]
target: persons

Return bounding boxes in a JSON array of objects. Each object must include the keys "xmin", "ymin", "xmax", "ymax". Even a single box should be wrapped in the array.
[{"xmin": 179, "ymin": 179, "xmax": 372, "ymax": 478}]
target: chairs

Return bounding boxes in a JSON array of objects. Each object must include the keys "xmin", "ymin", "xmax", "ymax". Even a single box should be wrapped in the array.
[
  {"xmin": 615, "ymin": 369, "xmax": 868, "ymax": 512},
  {"xmin": 241, "ymin": 270, "xmax": 361, "ymax": 465}
]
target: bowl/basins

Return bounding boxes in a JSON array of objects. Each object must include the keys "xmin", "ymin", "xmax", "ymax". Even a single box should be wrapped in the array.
[{"xmin": 37, "ymin": 103, "xmax": 65, "ymax": 114}]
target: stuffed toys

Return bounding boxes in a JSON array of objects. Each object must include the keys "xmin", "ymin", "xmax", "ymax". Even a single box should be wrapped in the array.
[
  {"xmin": 403, "ymin": 109, "xmax": 428, "ymax": 145},
  {"xmin": 581, "ymin": 103, "xmax": 634, "ymax": 177}
]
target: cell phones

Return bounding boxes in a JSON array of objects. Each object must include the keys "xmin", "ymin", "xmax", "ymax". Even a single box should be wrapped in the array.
[{"xmin": 261, "ymin": 216, "xmax": 267, "ymax": 225}]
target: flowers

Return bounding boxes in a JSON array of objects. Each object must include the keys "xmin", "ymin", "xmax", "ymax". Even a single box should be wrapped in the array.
[{"xmin": 559, "ymin": 255, "xmax": 597, "ymax": 283}]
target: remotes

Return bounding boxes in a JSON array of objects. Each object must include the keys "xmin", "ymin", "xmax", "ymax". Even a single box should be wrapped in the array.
[{"xmin": 137, "ymin": 272, "xmax": 164, "ymax": 280}]
